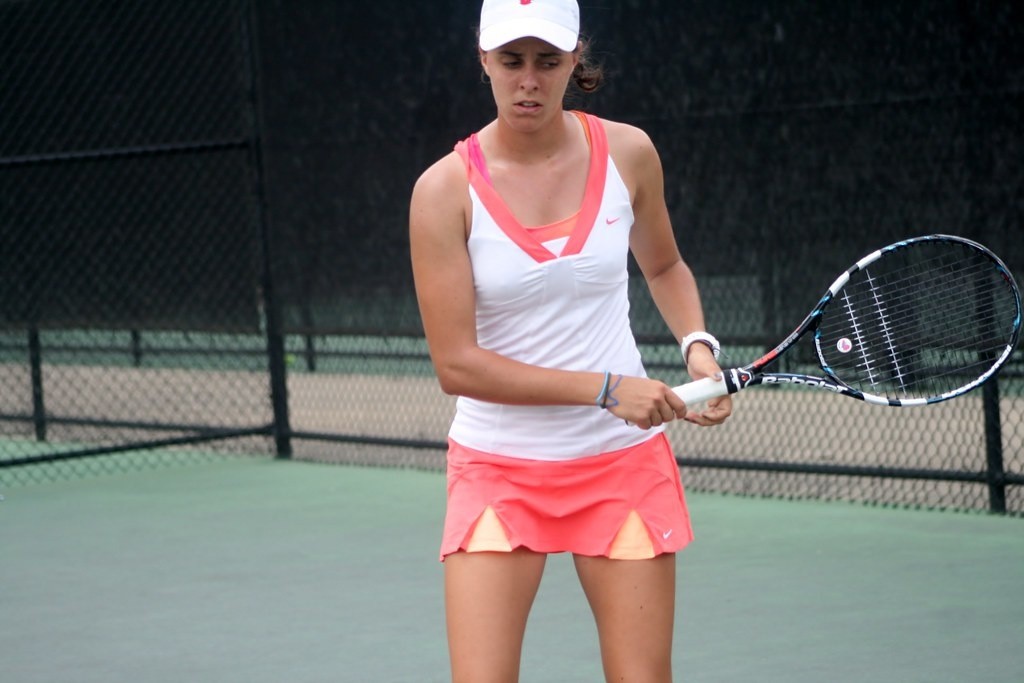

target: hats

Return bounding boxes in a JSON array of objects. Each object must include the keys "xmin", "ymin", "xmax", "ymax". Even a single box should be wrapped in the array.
[{"xmin": 479, "ymin": 0, "xmax": 579, "ymax": 53}]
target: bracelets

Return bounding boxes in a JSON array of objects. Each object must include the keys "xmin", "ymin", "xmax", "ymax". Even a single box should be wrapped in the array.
[
  {"xmin": 596, "ymin": 369, "xmax": 623, "ymax": 409},
  {"xmin": 680, "ymin": 331, "xmax": 720, "ymax": 363}
]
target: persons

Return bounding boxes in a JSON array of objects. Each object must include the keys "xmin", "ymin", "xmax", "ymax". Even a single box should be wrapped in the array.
[{"xmin": 407, "ymin": 0, "xmax": 734, "ymax": 683}]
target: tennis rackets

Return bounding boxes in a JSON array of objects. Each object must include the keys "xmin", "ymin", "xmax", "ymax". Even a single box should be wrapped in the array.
[{"xmin": 625, "ymin": 232, "xmax": 1022, "ymax": 428}]
